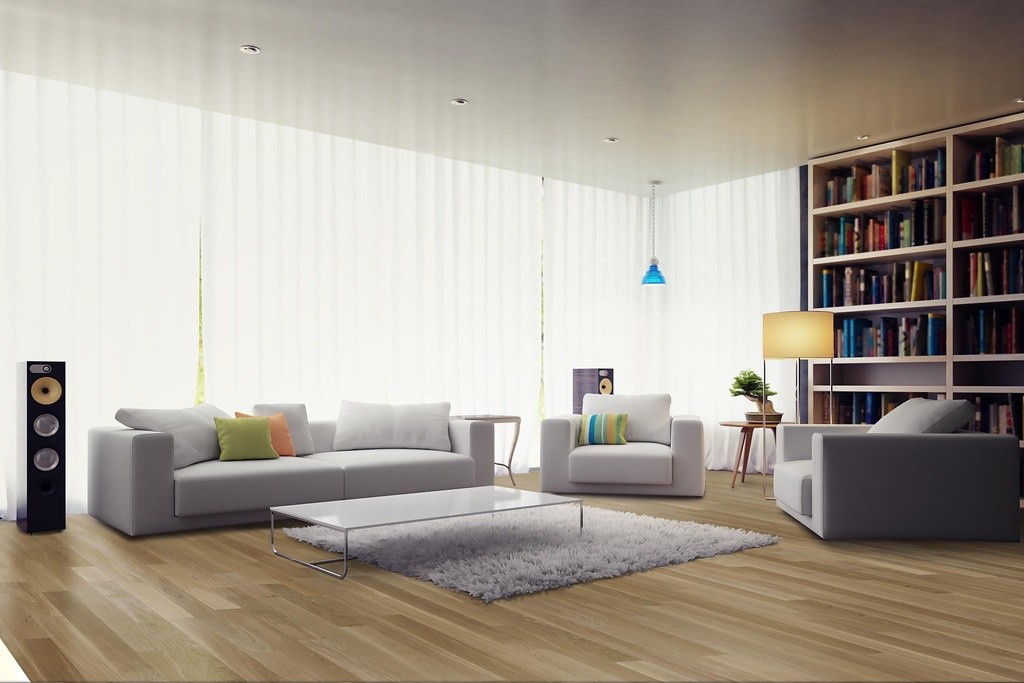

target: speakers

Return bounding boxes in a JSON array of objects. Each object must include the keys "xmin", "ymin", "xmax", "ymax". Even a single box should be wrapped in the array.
[
  {"xmin": 17, "ymin": 360, "xmax": 66, "ymax": 535},
  {"xmin": 573, "ymin": 368, "xmax": 614, "ymax": 414}
]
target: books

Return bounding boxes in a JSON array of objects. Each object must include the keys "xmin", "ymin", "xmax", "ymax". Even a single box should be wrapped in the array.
[{"xmin": 820, "ymin": 137, "xmax": 1024, "ymax": 435}]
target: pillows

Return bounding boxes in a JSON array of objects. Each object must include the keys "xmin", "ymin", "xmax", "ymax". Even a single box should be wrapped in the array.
[
  {"xmin": 868, "ymin": 398, "xmax": 975, "ymax": 433},
  {"xmin": 234, "ymin": 411, "xmax": 295, "ymax": 457},
  {"xmin": 576, "ymin": 414, "xmax": 628, "ymax": 446},
  {"xmin": 114, "ymin": 404, "xmax": 235, "ymax": 470},
  {"xmin": 213, "ymin": 416, "xmax": 280, "ymax": 462},
  {"xmin": 332, "ymin": 399, "xmax": 451, "ymax": 452},
  {"xmin": 254, "ymin": 404, "xmax": 314, "ymax": 456},
  {"xmin": 581, "ymin": 393, "xmax": 672, "ymax": 446}
]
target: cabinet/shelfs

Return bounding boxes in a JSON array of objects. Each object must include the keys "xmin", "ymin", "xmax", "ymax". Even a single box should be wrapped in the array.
[{"xmin": 808, "ymin": 112, "xmax": 1024, "ymax": 509}]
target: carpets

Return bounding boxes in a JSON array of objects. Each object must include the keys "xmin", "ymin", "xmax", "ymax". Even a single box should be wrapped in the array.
[{"xmin": 282, "ymin": 501, "xmax": 783, "ymax": 606}]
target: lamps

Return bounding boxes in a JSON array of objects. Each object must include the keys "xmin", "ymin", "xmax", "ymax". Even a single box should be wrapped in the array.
[
  {"xmin": 762, "ymin": 311, "xmax": 835, "ymax": 425},
  {"xmin": 640, "ymin": 181, "xmax": 666, "ymax": 284}
]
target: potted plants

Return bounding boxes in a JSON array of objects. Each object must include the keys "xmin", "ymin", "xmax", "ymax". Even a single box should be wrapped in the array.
[{"xmin": 729, "ymin": 369, "xmax": 785, "ymax": 424}]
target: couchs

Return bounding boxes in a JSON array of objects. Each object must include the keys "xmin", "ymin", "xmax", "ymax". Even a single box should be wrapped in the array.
[
  {"xmin": 774, "ymin": 424, "xmax": 1021, "ymax": 541},
  {"xmin": 540, "ymin": 395, "xmax": 705, "ymax": 497},
  {"xmin": 87, "ymin": 420, "xmax": 494, "ymax": 538}
]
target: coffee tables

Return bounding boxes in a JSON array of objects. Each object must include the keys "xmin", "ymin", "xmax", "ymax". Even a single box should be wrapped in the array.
[{"xmin": 269, "ymin": 485, "xmax": 584, "ymax": 581}]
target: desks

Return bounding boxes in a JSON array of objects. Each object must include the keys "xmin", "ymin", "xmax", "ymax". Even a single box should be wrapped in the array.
[
  {"xmin": 450, "ymin": 416, "xmax": 522, "ymax": 487},
  {"xmin": 719, "ymin": 421, "xmax": 796, "ymax": 488}
]
